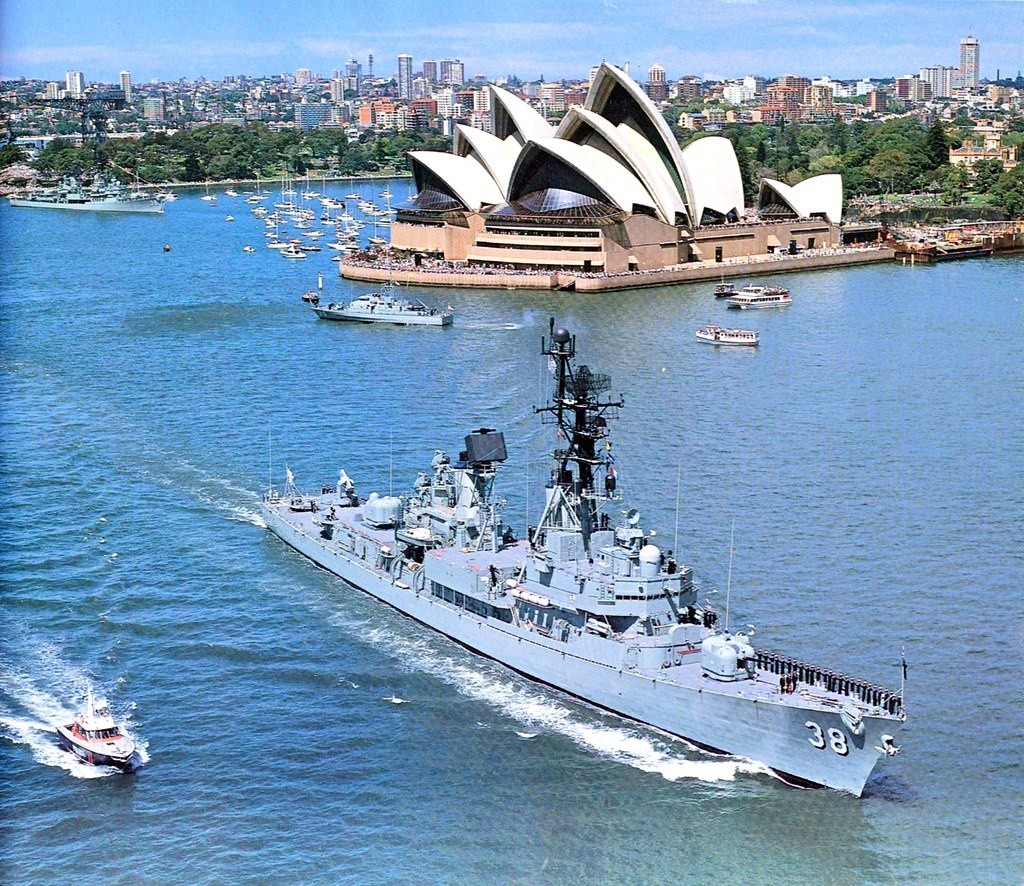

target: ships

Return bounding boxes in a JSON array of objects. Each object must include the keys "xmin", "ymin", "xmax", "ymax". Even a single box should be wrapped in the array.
[{"xmin": 251, "ymin": 317, "xmax": 906, "ymax": 799}]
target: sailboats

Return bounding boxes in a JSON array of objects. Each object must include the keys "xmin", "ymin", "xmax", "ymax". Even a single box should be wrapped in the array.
[
  {"xmin": 714, "ymin": 273, "xmax": 734, "ymax": 297},
  {"xmin": 200, "ymin": 167, "xmax": 418, "ymax": 251}
]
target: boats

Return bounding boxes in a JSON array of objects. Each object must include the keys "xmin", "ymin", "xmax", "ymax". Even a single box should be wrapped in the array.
[
  {"xmin": 55, "ymin": 691, "xmax": 136, "ymax": 772},
  {"xmin": 279, "ymin": 246, "xmax": 306, "ymax": 259},
  {"xmin": 695, "ymin": 324, "xmax": 760, "ymax": 346},
  {"xmin": 302, "ymin": 290, "xmax": 320, "ymax": 302},
  {"xmin": 310, "ymin": 269, "xmax": 454, "ymax": 324},
  {"xmin": 164, "ymin": 245, "xmax": 170, "ymax": 252},
  {"xmin": 725, "ymin": 284, "xmax": 792, "ymax": 311},
  {"xmin": 331, "ymin": 255, "xmax": 342, "ymax": 261},
  {"xmin": 6, "ymin": 172, "xmax": 176, "ymax": 214},
  {"xmin": 894, "ymin": 242, "xmax": 992, "ymax": 262},
  {"xmin": 299, "ymin": 244, "xmax": 322, "ymax": 251}
]
